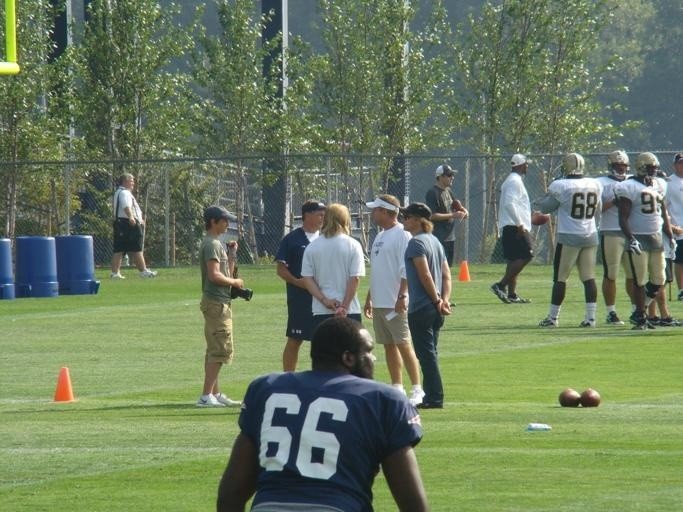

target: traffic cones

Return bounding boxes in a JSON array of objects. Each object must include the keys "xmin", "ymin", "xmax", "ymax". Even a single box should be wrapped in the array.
[
  {"xmin": 52, "ymin": 364, "xmax": 75, "ymax": 405},
  {"xmin": 456, "ymin": 258, "xmax": 472, "ymax": 282}
]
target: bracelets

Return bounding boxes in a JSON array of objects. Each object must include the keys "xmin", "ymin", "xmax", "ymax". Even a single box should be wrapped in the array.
[
  {"xmin": 340, "ymin": 303, "xmax": 351, "ymax": 310},
  {"xmin": 433, "ymin": 297, "xmax": 441, "ymax": 306}
]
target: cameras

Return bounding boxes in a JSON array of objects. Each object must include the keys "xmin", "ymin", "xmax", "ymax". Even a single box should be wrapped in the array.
[{"xmin": 231, "ymin": 286, "xmax": 253, "ymax": 301}]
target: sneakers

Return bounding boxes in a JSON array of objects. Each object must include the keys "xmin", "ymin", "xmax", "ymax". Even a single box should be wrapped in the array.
[
  {"xmin": 606, "ymin": 311, "xmax": 625, "ymax": 325},
  {"xmin": 138, "ymin": 268, "xmax": 158, "ymax": 278},
  {"xmin": 409, "ymin": 389, "xmax": 443, "ymax": 409},
  {"xmin": 507, "ymin": 294, "xmax": 532, "ymax": 303},
  {"xmin": 196, "ymin": 393, "xmax": 242, "ymax": 407},
  {"xmin": 538, "ymin": 314, "xmax": 558, "ymax": 328},
  {"xmin": 491, "ymin": 281, "xmax": 510, "ymax": 303},
  {"xmin": 629, "ymin": 312, "xmax": 683, "ymax": 330},
  {"xmin": 578, "ymin": 318, "xmax": 595, "ymax": 327},
  {"xmin": 110, "ymin": 269, "xmax": 125, "ymax": 279}
]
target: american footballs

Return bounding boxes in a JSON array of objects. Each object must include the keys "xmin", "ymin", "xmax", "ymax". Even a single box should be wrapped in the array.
[
  {"xmin": 531, "ymin": 211, "xmax": 551, "ymax": 225},
  {"xmin": 560, "ymin": 389, "xmax": 600, "ymax": 407}
]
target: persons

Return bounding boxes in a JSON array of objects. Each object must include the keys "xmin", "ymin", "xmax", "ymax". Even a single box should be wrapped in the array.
[
  {"xmin": 533, "ymin": 149, "xmax": 683, "ymax": 331},
  {"xmin": 364, "ymin": 194, "xmax": 426, "ymax": 408},
  {"xmin": 398, "ymin": 201, "xmax": 451, "ymax": 410},
  {"xmin": 271, "ymin": 198, "xmax": 328, "ymax": 373},
  {"xmin": 216, "ymin": 316, "xmax": 431, "ymax": 511},
  {"xmin": 489, "ymin": 154, "xmax": 535, "ymax": 304},
  {"xmin": 194, "ymin": 205, "xmax": 245, "ymax": 408},
  {"xmin": 110, "ymin": 172, "xmax": 159, "ymax": 278},
  {"xmin": 427, "ymin": 164, "xmax": 469, "ymax": 307},
  {"xmin": 301, "ymin": 203, "xmax": 366, "ymax": 326}
]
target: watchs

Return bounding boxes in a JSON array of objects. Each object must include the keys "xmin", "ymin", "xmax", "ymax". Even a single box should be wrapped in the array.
[{"xmin": 396, "ymin": 293, "xmax": 407, "ymax": 298}]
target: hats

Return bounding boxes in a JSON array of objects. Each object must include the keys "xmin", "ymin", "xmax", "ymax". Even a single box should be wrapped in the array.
[
  {"xmin": 511, "ymin": 153, "xmax": 533, "ymax": 167},
  {"xmin": 400, "ymin": 203, "xmax": 432, "ymax": 220},
  {"xmin": 673, "ymin": 152, "xmax": 682, "ymax": 163},
  {"xmin": 434, "ymin": 164, "xmax": 458, "ymax": 177},
  {"xmin": 366, "ymin": 196, "xmax": 400, "ymax": 213},
  {"xmin": 204, "ymin": 205, "xmax": 237, "ymax": 221},
  {"xmin": 302, "ymin": 203, "xmax": 326, "ymax": 214}
]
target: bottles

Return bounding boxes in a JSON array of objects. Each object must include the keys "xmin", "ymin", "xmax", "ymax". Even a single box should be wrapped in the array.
[{"xmin": 528, "ymin": 423, "xmax": 552, "ymax": 431}]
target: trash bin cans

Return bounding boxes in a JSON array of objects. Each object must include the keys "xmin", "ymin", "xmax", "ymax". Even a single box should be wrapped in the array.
[{"xmin": 0, "ymin": 235, "xmax": 100, "ymax": 299}]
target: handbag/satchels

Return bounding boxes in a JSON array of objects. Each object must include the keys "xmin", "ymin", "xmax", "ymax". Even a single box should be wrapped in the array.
[{"xmin": 113, "ymin": 217, "xmax": 143, "ymax": 244}]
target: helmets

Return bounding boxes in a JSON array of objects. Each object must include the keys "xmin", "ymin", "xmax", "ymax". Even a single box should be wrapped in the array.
[
  {"xmin": 607, "ymin": 150, "xmax": 629, "ymax": 179},
  {"xmin": 636, "ymin": 152, "xmax": 660, "ymax": 176},
  {"xmin": 560, "ymin": 152, "xmax": 585, "ymax": 176}
]
